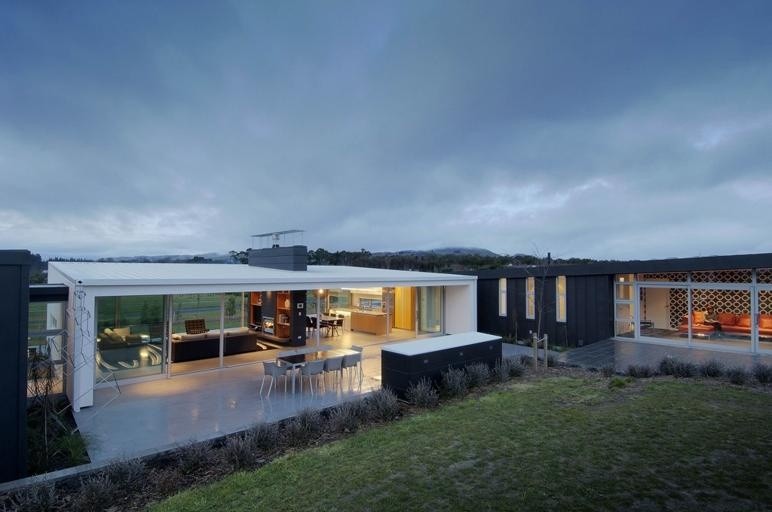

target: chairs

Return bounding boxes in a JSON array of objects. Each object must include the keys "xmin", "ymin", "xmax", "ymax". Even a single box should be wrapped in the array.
[
  {"xmin": 306, "ymin": 313, "xmax": 344, "ymax": 337},
  {"xmin": 260, "ymin": 343, "xmax": 363, "ymax": 397}
]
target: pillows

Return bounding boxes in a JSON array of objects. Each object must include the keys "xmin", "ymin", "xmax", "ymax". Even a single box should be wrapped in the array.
[{"xmin": 113, "ymin": 327, "xmax": 131, "ymax": 337}]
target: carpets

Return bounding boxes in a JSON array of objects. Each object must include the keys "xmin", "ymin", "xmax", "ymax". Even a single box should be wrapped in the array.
[{"xmin": 95, "ymin": 339, "xmax": 175, "ymax": 372}]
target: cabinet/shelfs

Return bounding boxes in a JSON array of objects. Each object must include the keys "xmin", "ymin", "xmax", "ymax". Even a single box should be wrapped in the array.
[
  {"xmin": 382, "ymin": 331, "xmax": 503, "ymax": 403},
  {"xmin": 250, "ymin": 290, "xmax": 293, "ymax": 338}
]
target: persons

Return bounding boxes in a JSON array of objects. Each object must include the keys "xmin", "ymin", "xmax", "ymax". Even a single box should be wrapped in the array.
[{"xmin": 704, "ymin": 305, "xmax": 722, "ymax": 337}]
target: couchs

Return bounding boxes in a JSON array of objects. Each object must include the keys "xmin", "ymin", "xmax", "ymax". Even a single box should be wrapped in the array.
[
  {"xmin": 679, "ymin": 310, "xmax": 771, "ymax": 341},
  {"xmin": 98, "ymin": 327, "xmax": 148, "ymax": 362},
  {"xmin": 173, "ymin": 327, "xmax": 260, "ymax": 360}
]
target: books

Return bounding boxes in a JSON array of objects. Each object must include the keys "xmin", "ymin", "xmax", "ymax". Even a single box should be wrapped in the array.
[{"xmin": 709, "ymin": 319, "xmax": 718, "ymax": 323}]
